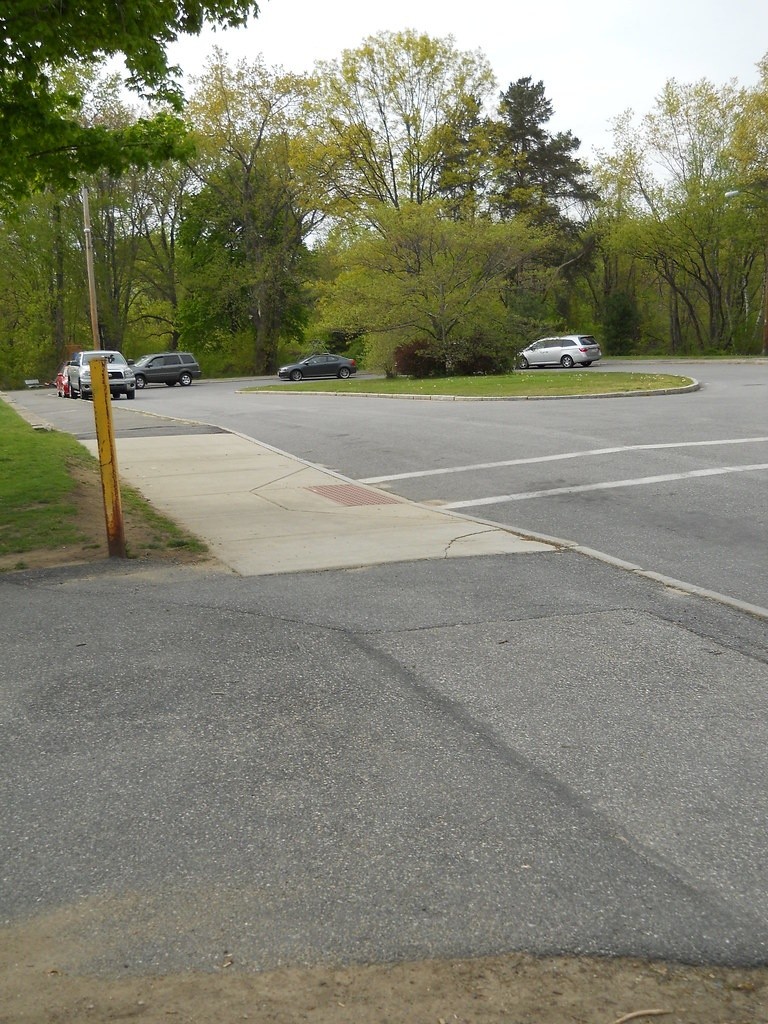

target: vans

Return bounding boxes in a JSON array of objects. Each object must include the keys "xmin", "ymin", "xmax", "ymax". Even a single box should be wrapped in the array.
[{"xmin": 515, "ymin": 335, "xmax": 601, "ymax": 368}]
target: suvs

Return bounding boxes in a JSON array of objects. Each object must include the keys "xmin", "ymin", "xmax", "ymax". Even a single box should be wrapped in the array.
[
  {"xmin": 128, "ymin": 353, "xmax": 201, "ymax": 387},
  {"xmin": 66, "ymin": 351, "xmax": 136, "ymax": 400},
  {"xmin": 25, "ymin": 378, "xmax": 42, "ymax": 389}
]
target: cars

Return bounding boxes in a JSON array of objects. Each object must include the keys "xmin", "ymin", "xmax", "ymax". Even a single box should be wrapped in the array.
[
  {"xmin": 56, "ymin": 364, "xmax": 69, "ymax": 398},
  {"xmin": 277, "ymin": 352, "xmax": 356, "ymax": 381},
  {"xmin": 45, "ymin": 379, "xmax": 56, "ymax": 388}
]
